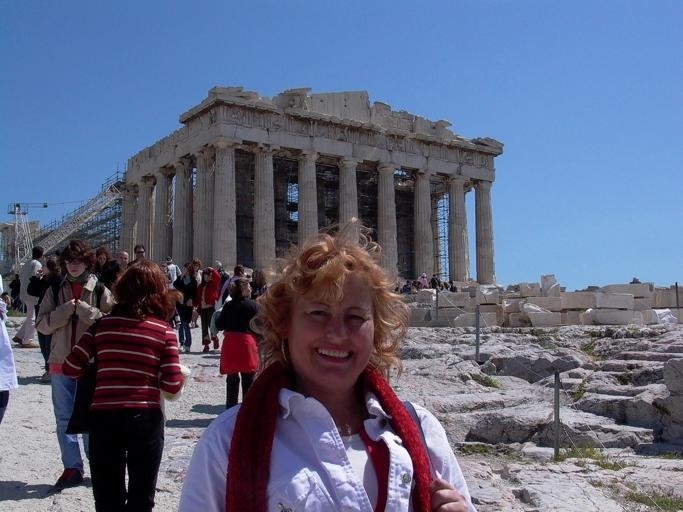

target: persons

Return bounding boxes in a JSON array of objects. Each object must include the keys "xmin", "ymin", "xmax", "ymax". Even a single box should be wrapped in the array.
[
  {"xmin": 389, "ymin": 273, "xmax": 457, "ymax": 296},
  {"xmin": 179, "ymin": 217, "xmax": 474, "ymax": 511},
  {"xmin": 0, "ymin": 239, "xmax": 267, "ymax": 512}
]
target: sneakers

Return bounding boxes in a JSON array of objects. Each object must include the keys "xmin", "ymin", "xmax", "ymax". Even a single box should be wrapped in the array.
[
  {"xmin": 178, "ymin": 346, "xmax": 184, "ymax": 351},
  {"xmin": 214, "ymin": 338, "xmax": 219, "ymax": 349},
  {"xmin": 203, "ymin": 346, "xmax": 209, "ymax": 353},
  {"xmin": 180, "ymin": 346, "xmax": 190, "ymax": 353},
  {"xmin": 54, "ymin": 468, "xmax": 83, "ymax": 490},
  {"xmin": 41, "ymin": 371, "xmax": 50, "ymax": 380},
  {"xmin": 12, "ymin": 336, "xmax": 22, "ymax": 344}
]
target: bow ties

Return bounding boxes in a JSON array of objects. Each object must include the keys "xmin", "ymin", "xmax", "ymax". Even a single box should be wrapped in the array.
[{"xmin": 22, "ymin": 341, "xmax": 40, "ymax": 348}]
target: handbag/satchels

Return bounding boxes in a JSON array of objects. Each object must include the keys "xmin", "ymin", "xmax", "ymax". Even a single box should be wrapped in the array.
[{"xmin": 66, "ymin": 316, "xmax": 104, "ymax": 434}]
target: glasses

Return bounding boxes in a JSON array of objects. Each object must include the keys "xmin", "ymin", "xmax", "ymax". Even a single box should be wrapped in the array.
[
  {"xmin": 62, "ymin": 257, "xmax": 81, "ymax": 265},
  {"xmin": 136, "ymin": 250, "xmax": 144, "ymax": 254}
]
